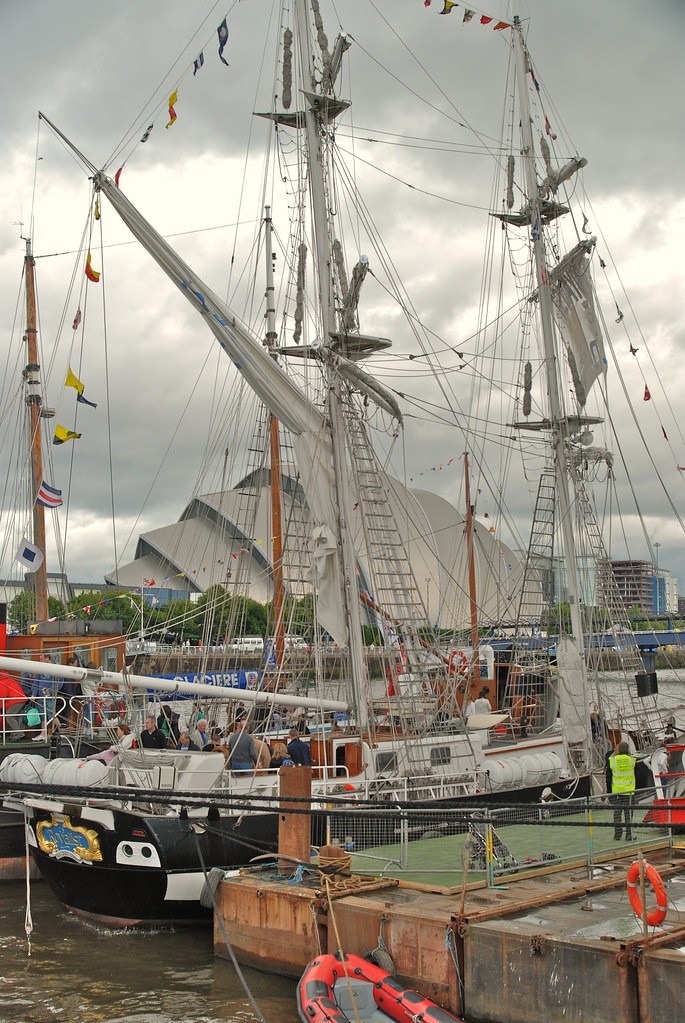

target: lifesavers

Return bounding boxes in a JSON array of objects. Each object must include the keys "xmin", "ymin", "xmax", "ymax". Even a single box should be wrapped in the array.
[
  {"xmin": 449, "ymin": 651, "xmax": 467, "ymax": 672},
  {"xmin": 363, "ymin": 950, "xmax": 396, "ymax": 976},
  {"xmin": 327, "ymin": 783, "xmax": 358, "ymax": 809},
  {"xmin": 626, "ymin": 861, "xmax": 669, "ymax": 926},
  {"xmin": 199, "ymin": 866, "xmax": 225, "ymax": 908},
  {"xmin": 95, "ymin": 691, "xmax": 126, "ymax": 727}
]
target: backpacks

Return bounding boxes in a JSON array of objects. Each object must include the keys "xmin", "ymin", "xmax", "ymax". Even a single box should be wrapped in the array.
[{"xmin": 281, "ymin": 758, "xmax": 294, "ymax": 766}]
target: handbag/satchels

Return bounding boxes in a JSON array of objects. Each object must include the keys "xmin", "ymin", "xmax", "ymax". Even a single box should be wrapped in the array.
[{"xmin": 22, "ymin": 700, "xmax": 41, "ymax": 727}]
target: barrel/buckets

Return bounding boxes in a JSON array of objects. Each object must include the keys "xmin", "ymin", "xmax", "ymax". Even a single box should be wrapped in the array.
[{"xmin": 635, "ymin": 671, "xmax": 658, "ymax": 697}]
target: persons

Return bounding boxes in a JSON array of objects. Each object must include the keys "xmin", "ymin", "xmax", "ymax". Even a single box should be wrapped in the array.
[
  {"xmin": 80, "ymin": 662, "xmax": 98, "ymax": 735},
  {"xmin": 474, "ymin": 690, "xmax": 492, "ymax": 746},
  {"xmin": 186, "ymin": 703, "xmax": 207, "ymax": 731},
  {"xmin": 228, "ymin": 721, "xmax": 262, "ymax": 776},
  {"xmin": 235, "ymin": 701, "xmax": 248, "ymax": 722},
  {"xmin": 0, "ymin": 648, "xmax": 497, "ymax": 821},
  {"xmin": 175, "ymin": 732, "xmax": 201, "ymax": 751},
  {"xmin": 462, "ymin": 692, "xmax": 474, "ymax": 727},
  {"xmin": 157, "ymin": 705, "xmax": 181, "ymax": 749},
  {"xmin": 287, "ymin": 729, "xmax": 312, "ymax": 766},
  {"xmin": 0, "ymin": 669, "xmax": 28, "ymax": 742},
  {"xmin": 141, "ymin": 716, "xmax": 167, "ymax": 749},
  {"xmin": 110, "ymin": 723, "xmax": 135, "ymax": 756},
  {"xmin": 31, "ymin": 654, "xmax": 65, "ymax": 740},
  {"xmin": 190, "ymin": 719, "xmax": 209, "ymax": 751},
  {"xmin": 268, "ymin": 743, "xmax": 294, "ymax": 776},
  {"xmin": 604, "ymin": 741, "xmax": 639, "ymax": 841}
]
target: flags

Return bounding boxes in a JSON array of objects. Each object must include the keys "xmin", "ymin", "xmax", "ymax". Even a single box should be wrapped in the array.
[
  {"xmin": 480, "ymin": 14, "xmax": 492, "ymax": 25},
  {"xmin": 35, "ymin": 480, "xmax": 63, "ymax": 509},
  {"xmin": 493, "ymin": 21, "xmax": 511, "ymax": 32},
  {"xmin": 83, "ymin": 250, "xmax": 102, "ymax": 283},
  {"xmin": 15, "ymin": 538, "xmax": 44, "ymax": 574},
  {"xmin": 143, "ymin": 577, "xmax": 156, "ymax": 588},
  {"xmin": 52, "ymin": 423, "xmax": 83, "ymax": 446},
  {"xmin": 461, "ymin": 8, "xmax": 476, "ymax": 24}
]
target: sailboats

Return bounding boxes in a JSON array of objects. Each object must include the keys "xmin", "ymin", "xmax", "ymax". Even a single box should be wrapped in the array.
[{"xmin": 0, "ymin": 0, "xmax": 685, "ymax": 929}]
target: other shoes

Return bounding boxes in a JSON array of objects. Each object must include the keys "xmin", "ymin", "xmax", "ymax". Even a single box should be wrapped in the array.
[
  {"xmin": 32, "ymin": 734, "xmax": 51, "ymax": 741},
  {"xmin": 625, "ymin": 836, "xmax": 637, "ymax": 840},
  {"xmin": 82, "ymin": 728, "xmax": 98, "ymax": 736}
]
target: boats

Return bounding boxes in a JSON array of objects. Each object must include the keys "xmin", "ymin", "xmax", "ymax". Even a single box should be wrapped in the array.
[{"xmin": 295, "ymin": 951, "xmax": 465, "ymax": 1023}]
